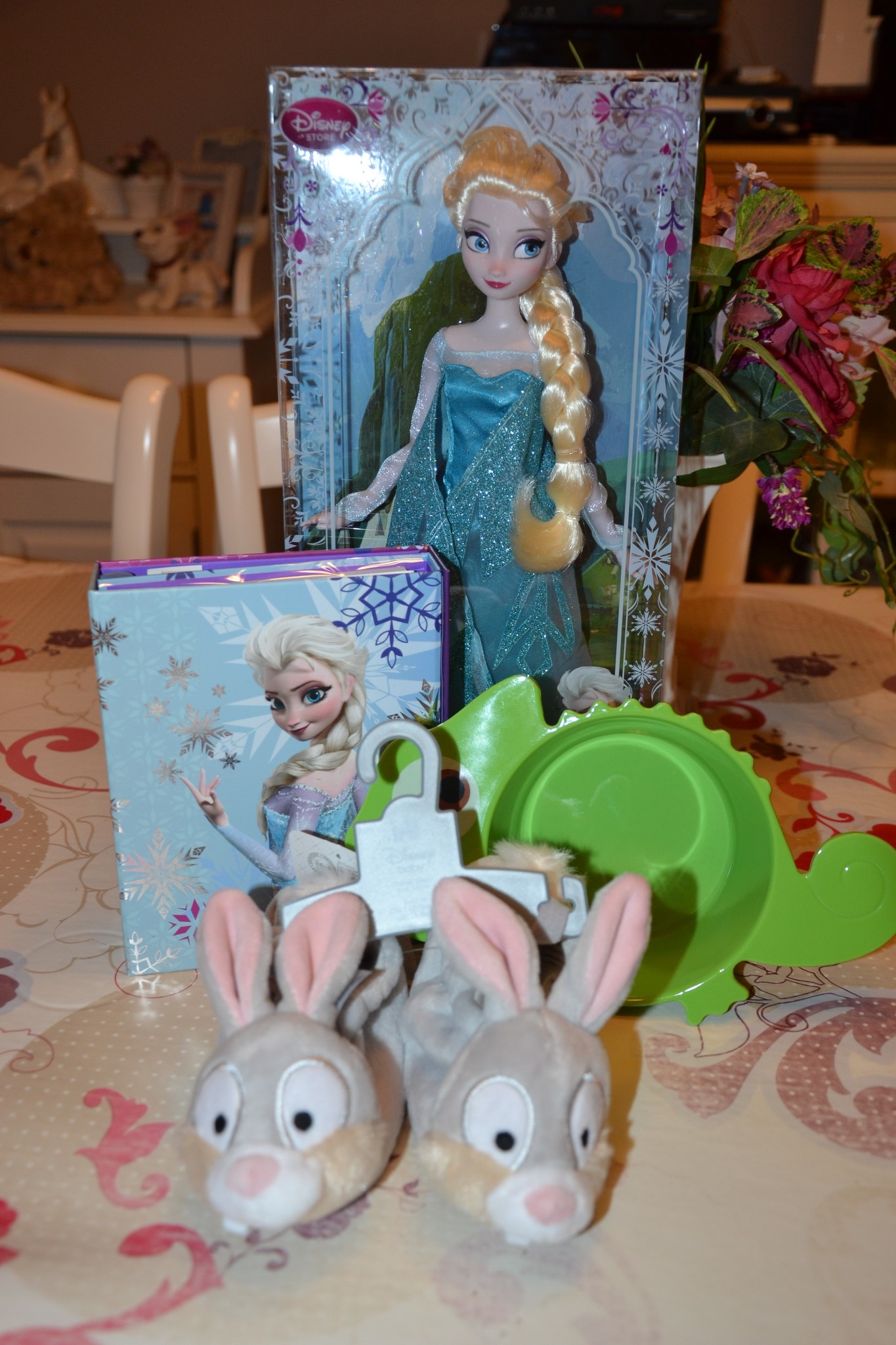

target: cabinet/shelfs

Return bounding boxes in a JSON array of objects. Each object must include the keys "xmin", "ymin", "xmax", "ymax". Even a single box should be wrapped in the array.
[{"xmin": 3, "ymin": 302, "xmax": 279, "ymax": 570}]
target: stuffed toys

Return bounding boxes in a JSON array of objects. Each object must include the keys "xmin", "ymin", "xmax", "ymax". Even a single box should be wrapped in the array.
[
  {"xmin": 396, "ymin": 836, "xmax": 666, "ymax": 1237},
  {"xmin": 183, "ymin": 866, "xmax": 427, "ymax": 1246},
  {"xmin": 0, "ymin": 174, "xmax": 126, "ymax": 314}
]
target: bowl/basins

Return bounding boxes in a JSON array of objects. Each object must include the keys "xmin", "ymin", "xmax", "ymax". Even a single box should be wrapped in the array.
[{"xmin": 345, "ymin": 673, "xmax": 896, "ymax": 1024}]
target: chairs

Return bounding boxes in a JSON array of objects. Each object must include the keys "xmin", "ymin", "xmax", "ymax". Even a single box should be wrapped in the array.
[
  {"xmin": 3, "ymin": 368, "xmax": 183, "ymax": 568},
  {"xmin": 205, "ymin": 378, "xmax": 294, "ymax": 563}
]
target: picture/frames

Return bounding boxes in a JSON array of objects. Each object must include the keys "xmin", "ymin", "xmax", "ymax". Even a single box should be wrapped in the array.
[
  {"xmin": 265, "ymin": 63, "xmax": 705, "ymax": 715},
  {"xmin": 189, "ymin": 124, "xmax": 269, "ymax": 223},
  {"xmin": 165, "ymin": 161, "xmax": 240, "ymax": 292}
]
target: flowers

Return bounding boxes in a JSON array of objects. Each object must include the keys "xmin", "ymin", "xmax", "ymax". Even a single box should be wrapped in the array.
[
  {"xmin": 101, "ymin": 134, "xmax": 175, "ymax": 183},
  {"xmin": 667, "ymin": 128, "xmax": 896, "ymax": 609}
]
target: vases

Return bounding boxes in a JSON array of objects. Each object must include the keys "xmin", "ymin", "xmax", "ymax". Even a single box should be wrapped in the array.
[
  {"xmin": 659, "ymin": 446, "xmax": 730, "ymax": 705},
  {"xmin": 120, "ymin": 174, "xmax": 167, "ymax": 222}
]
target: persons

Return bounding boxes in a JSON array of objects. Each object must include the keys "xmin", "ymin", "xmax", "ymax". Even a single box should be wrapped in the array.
[{"xmin": 300, "ymin": 127, "xmax": 664, "ymax": 730}]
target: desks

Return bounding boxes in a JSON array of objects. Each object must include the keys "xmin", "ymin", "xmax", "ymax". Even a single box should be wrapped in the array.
[{"xmin": 0, "ymin": 556, "xmax": 894, "ymax": 1343}]
